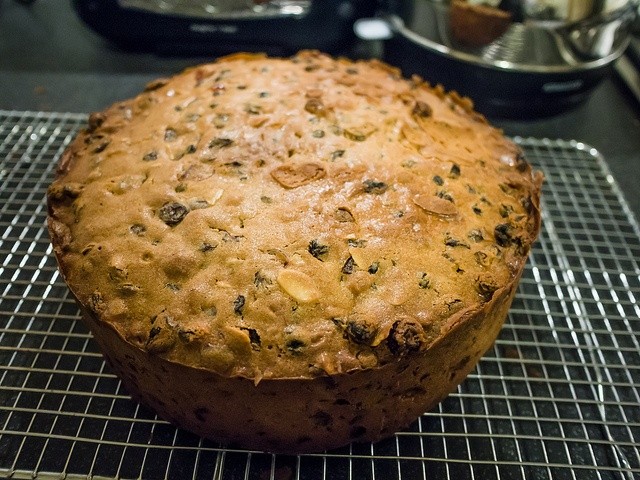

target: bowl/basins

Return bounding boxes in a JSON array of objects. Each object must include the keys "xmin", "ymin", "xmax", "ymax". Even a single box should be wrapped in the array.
[{"xmin": 389, "ymin": 1, "xmax": 631, "ymax": 122}]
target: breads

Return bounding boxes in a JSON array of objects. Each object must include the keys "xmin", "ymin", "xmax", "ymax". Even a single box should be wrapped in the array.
[{"xmin": 46, "ymin": 48, "xmax": 542, "ymax": 454}]
